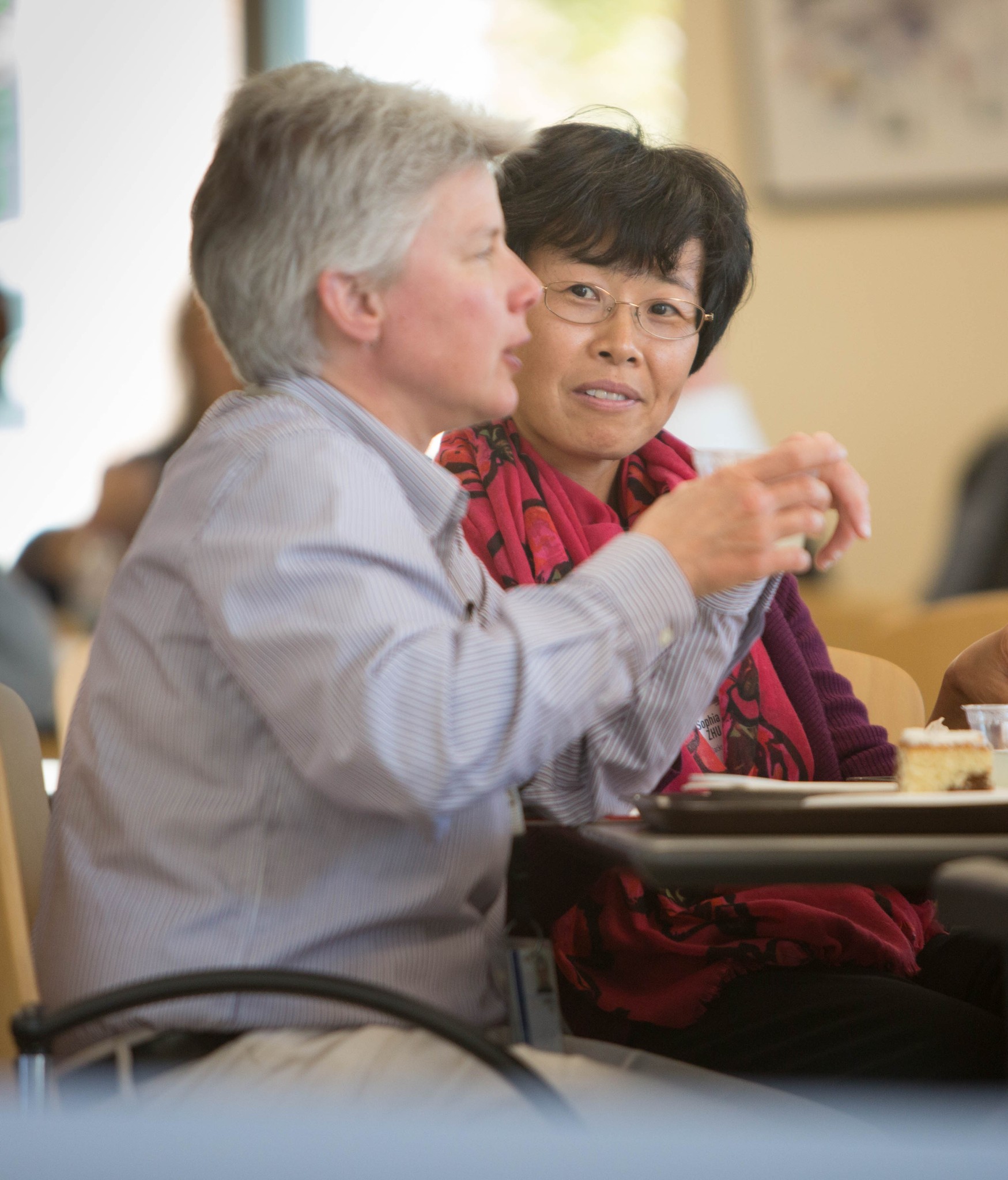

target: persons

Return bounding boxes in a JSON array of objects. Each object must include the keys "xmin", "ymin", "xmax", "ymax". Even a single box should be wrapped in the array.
[
  {"xmin": 38, "ymin": 63, "xmax": 904, "ymax": 1176},
  {"xmin": 435, "ymin": 119, "xmax": 1008, "ymax": 1080}
]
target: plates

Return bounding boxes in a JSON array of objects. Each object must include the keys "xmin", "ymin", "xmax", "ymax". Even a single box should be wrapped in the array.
[{"xmin": 685, "ymin": 772, "xmax": 1008, "ymax": 806}]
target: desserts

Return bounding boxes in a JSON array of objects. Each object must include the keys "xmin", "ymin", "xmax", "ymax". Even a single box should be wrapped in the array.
[{"xmin": 896, "ymin": 718, "xmax": 993, "ymax": 794}]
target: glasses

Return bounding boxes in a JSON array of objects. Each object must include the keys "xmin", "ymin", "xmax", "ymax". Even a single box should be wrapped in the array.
[{"xmin": 542, "ymin": 280, "xmax": 715, "ymax": 341}]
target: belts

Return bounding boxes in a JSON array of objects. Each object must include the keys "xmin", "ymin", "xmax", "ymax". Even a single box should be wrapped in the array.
[{"xmin": 56, "ymin": 1028, "xmax": 244, "ymax": 1113}]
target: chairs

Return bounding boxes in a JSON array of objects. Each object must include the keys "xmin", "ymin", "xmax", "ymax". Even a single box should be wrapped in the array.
[{"xmin": 0, "ymin": 677, "xmax": 614, "ymax": 1127}]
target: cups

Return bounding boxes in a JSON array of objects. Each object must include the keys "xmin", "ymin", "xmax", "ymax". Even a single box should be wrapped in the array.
[{"xmin": 961, "ymin": 703, "xmax": 1008, "ymax": 792}]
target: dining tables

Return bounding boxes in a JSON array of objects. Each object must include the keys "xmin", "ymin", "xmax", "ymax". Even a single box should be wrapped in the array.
[{"xmin": 574, "ymin": 809, "xmax": 1008, "ymax": 890}]
